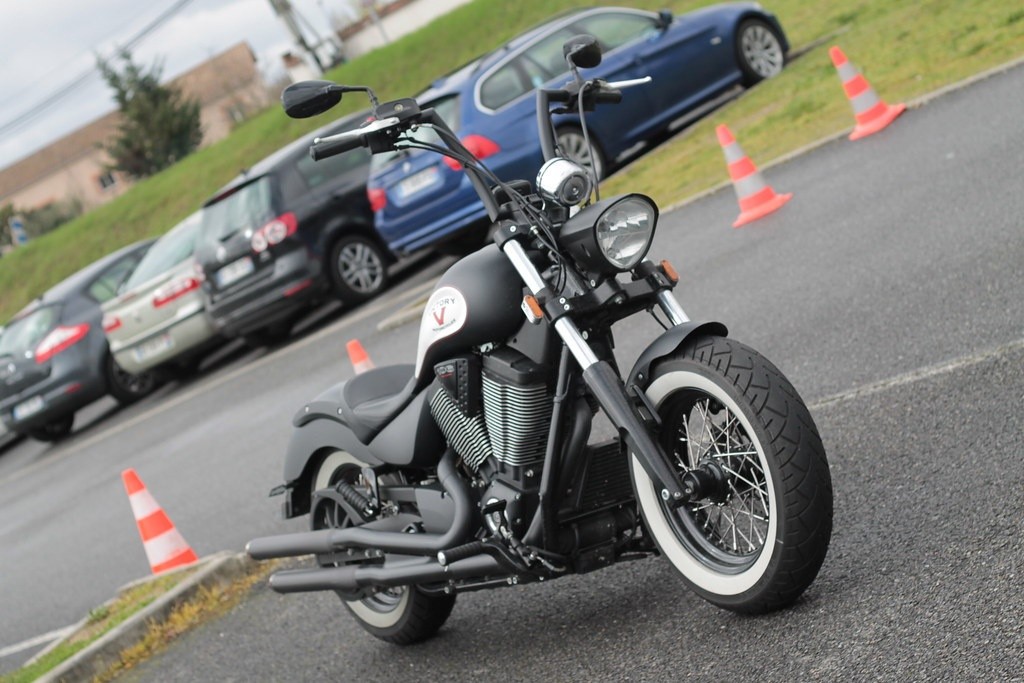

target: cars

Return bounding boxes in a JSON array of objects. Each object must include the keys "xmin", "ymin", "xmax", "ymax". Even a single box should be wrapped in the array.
[
  {"xmin": 367, "ymin": 7, "xmax": 790, "ymax": 262},
  {"xmin": 0, "ymin": 236, "xmax": 157, "ymax": 442},
  {"xmin": 98, "ymin": 206, "xmax": 216, "ymax": 385}
]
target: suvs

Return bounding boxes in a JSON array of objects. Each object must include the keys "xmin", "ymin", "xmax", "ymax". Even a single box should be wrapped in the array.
[{"xmin": 195, "ymin": 104, "xmax": 399, "ymax": 350}]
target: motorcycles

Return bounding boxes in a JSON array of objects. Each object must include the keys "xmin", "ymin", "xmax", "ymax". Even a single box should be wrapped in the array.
[{"xmin": 244, "ymin": 33, "xmax": 833, "ymax": 642}]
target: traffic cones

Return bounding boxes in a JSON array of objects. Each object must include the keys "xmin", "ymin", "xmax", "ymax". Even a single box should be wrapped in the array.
[
  {"xmin": 121, "ymin": 468, "xmax": 199, "ymax": 575},
  {"xmin": 343, "ymin": 337, "xmax": 375, "ymax": 377},
  {"xmin": 827, "ymin": 45, "xmax": 905, "ymax": 143},
  {"xmin": 713, "ymin": 123, "xmax": 792, "ymax": 229}
]
